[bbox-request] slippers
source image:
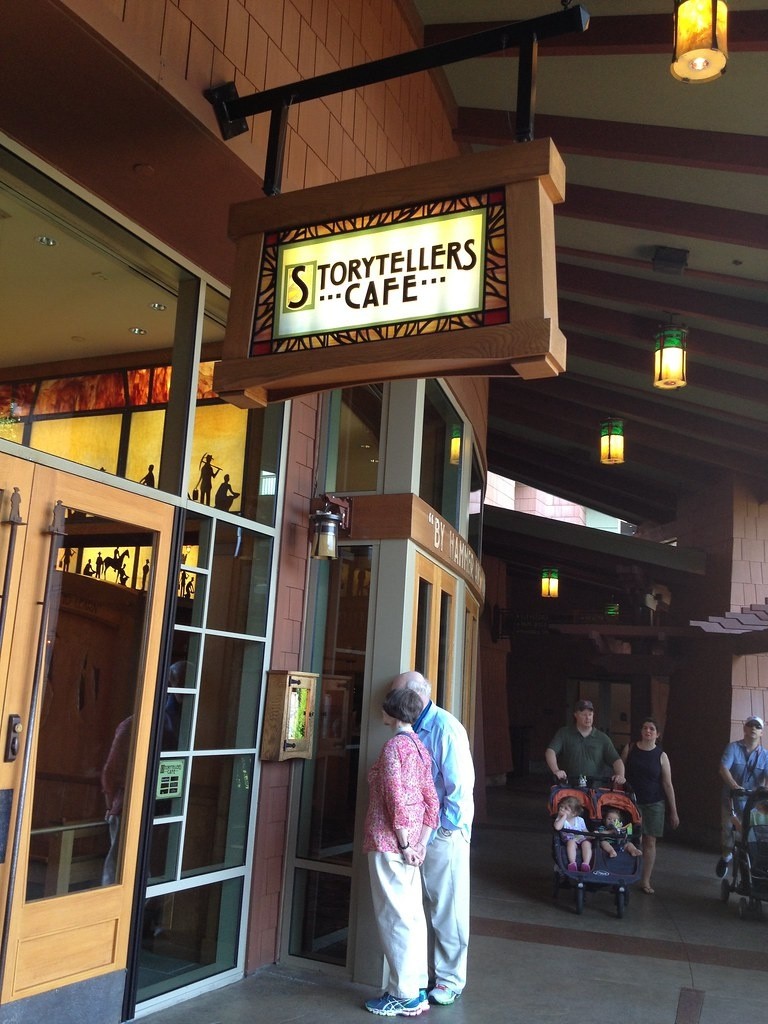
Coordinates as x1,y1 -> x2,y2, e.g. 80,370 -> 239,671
642,883 -> 655,896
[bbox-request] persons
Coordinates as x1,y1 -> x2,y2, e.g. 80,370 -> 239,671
599,810 -> 642,857
545,700 -> 626,789
615,717 -> 680,894
553,796 -> 592,872
392,671 -> 476,1004
716,716 -> 768,877
99,654 -> 201,941
730,787 -> 768,830
361,688 -> 440,1017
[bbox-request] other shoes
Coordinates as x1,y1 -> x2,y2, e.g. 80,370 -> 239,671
580,862 -> 591,873
568,862 -> 577,872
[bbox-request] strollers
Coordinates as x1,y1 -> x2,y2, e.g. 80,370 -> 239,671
548,772 -> 643,919
722,786 -> 767,921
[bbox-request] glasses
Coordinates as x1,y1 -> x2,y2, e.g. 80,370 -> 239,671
745,722 -> 762,729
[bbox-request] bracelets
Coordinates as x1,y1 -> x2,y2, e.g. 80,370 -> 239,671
398,842 -> 409,849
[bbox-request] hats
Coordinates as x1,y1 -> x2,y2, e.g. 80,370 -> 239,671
745,716 -> 764,729
575,700 -> 594,712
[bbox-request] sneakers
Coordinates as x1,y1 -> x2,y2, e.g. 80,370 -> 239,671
363,983 -> 431,1016
429,982 -> 461,1005
716,853 -> 731,878
